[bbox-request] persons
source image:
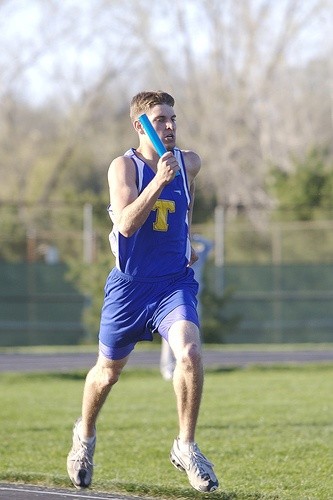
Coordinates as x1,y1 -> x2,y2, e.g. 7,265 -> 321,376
63,92 -> 222,493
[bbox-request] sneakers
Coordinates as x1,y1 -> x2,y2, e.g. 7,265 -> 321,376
169,437 -> 219,492
66,420 -> 96,489
